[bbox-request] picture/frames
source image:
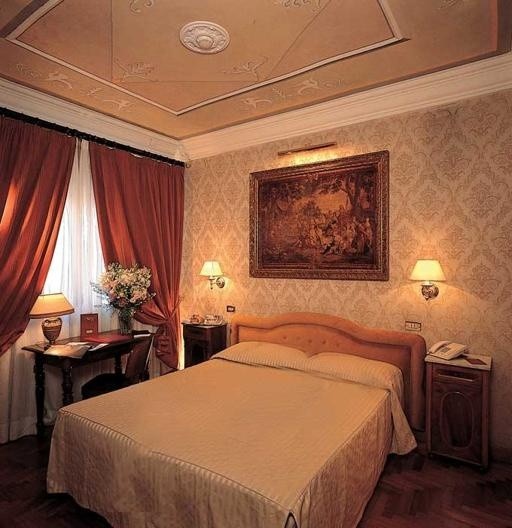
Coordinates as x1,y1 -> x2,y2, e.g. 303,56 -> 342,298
247,149 -> 391,283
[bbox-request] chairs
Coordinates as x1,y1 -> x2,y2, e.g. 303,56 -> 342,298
80,336 -> 153,399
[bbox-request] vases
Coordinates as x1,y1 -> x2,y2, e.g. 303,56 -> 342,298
116,309 -> 136,334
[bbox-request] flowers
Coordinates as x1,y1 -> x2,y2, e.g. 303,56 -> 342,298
88,262 -> 158,333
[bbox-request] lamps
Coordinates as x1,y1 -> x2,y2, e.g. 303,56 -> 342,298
28,291 -> 72,345
408,259 -> 447,302
200,260 -> 227,290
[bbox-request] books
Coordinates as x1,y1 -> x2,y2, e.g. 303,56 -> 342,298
131,330 -> 152,338
43,341 -> 109,359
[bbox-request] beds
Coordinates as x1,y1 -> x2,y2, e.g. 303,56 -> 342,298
57,310 -> 425,528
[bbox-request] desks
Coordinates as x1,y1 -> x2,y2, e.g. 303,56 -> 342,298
21,327 -> 153,434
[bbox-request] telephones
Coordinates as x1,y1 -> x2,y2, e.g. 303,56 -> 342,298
428,341 -> 467,360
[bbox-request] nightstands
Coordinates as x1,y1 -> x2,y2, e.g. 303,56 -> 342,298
181,321 -> 228,369
424,351 -> 494,472
